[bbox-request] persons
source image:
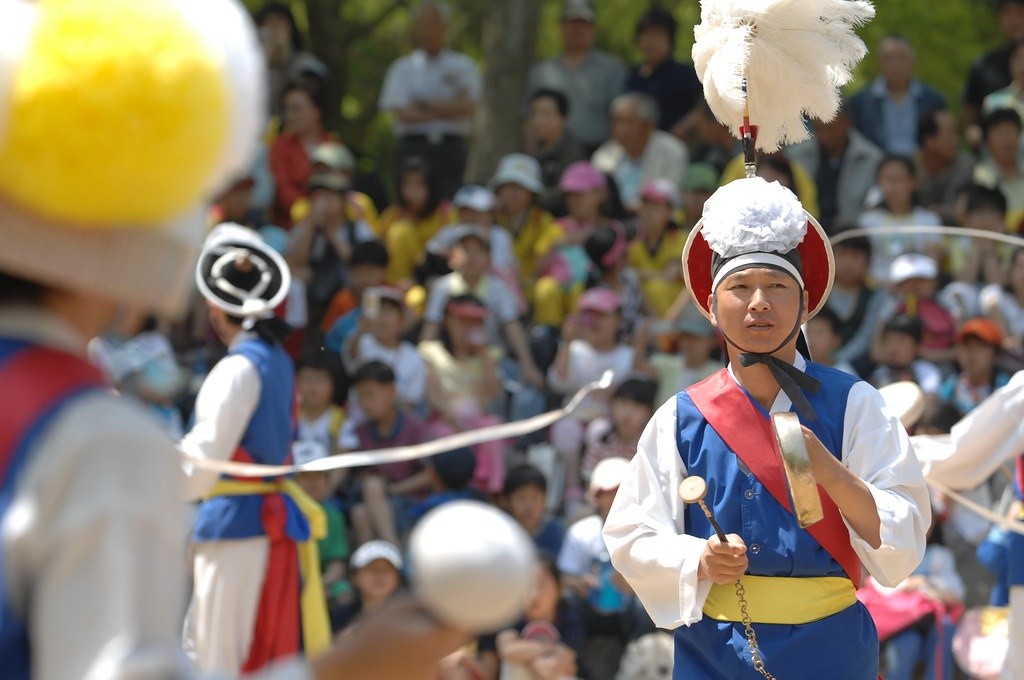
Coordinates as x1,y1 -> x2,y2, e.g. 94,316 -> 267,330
175,222 -> 310,675
90,0 -> 1024,680
600,177 -> 933,680
0,0 -> 479,680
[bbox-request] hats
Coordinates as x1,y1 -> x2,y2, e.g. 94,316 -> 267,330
196,222 -> 291,329
453,184 -> 495,212
890,254 -> 937,284
589,457 -> 630,498
559,161 -> 609,195
640,177 -> 680,212
680,0 -> 875,321
958,317 -> 1004,347
350,540 -> 403,571
0,0 -> 263,319
490,154 -> 544,199
579,290 -> 622,314
563,0 -> 599,26
885,312 -> 923,342
